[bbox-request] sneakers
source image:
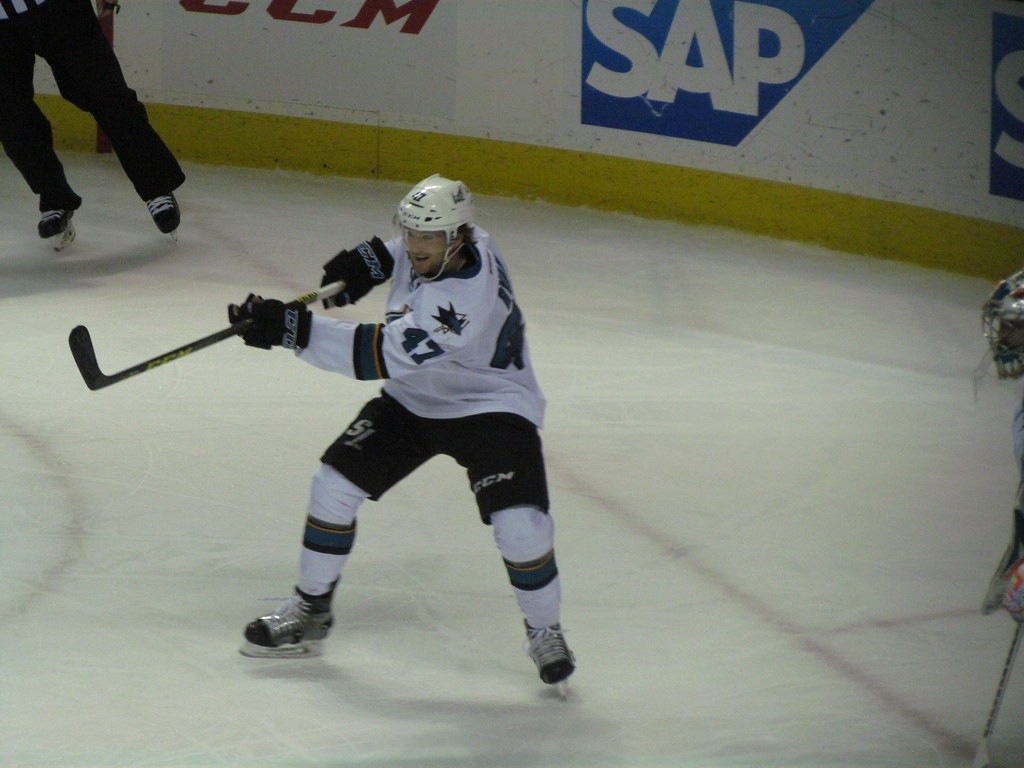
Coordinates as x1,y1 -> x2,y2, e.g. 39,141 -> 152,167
37,208 -> 76,252
239,577 -> 340,659
145,193 -> 181,242
521,620 -> 575,699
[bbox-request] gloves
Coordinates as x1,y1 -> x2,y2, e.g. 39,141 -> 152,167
229,293 -> 313,350
998,541 -> 1024,629
321,234 -> 394,309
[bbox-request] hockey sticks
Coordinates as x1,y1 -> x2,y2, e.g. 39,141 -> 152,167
972,622 -> 1023,768
67,278 -> 350,394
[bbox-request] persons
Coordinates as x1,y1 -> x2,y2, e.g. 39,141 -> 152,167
228,174 -> 575,700
0,0 -> 185,253
981,272 -> 1024,623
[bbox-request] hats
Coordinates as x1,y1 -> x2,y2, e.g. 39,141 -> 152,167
393,173 -> 476,262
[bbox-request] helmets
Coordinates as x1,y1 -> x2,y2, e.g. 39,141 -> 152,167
981,269 -> 1024,379
